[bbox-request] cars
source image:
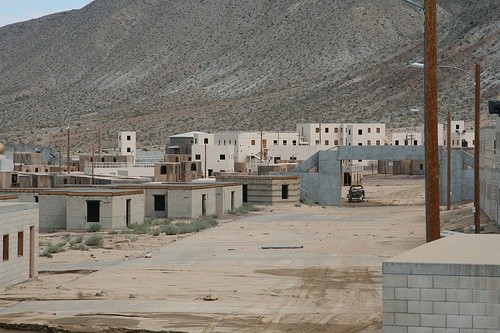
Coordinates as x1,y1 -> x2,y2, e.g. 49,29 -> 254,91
348,184 -> 365,198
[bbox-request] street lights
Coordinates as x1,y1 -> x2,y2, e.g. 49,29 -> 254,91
410,106 -> 453,212
407,61 -> 484,234
